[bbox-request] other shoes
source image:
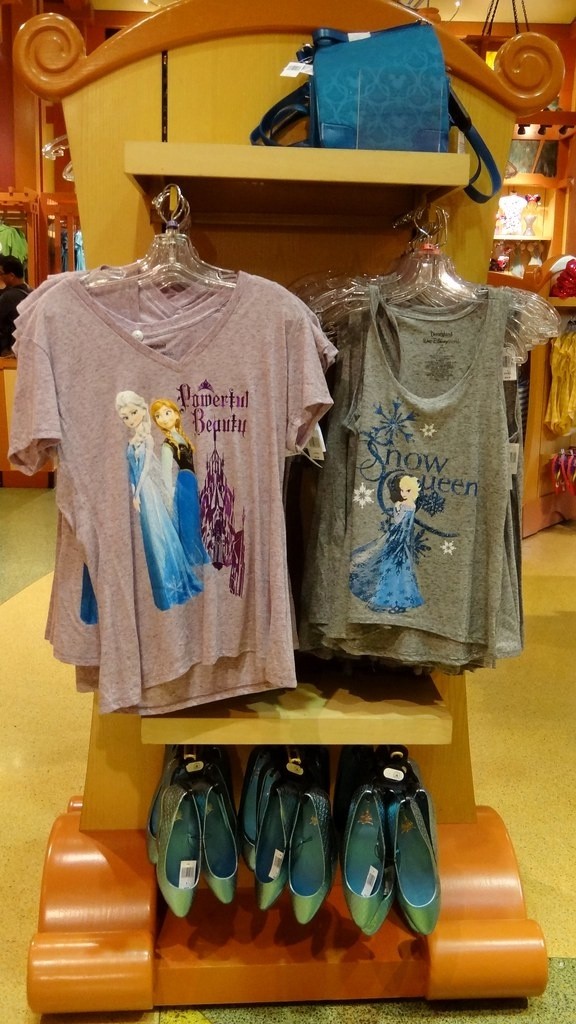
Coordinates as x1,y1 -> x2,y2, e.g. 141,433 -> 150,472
147,746 -> 443,939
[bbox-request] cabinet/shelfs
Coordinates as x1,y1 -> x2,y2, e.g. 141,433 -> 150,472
120,138 -> 472,751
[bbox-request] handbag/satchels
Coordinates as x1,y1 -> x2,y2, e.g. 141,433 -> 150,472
251,18 -> 502,203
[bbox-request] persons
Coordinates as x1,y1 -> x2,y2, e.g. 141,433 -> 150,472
1,254 -> 36,359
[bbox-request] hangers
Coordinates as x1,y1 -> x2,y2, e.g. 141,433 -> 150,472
76,182 -> 568,367
